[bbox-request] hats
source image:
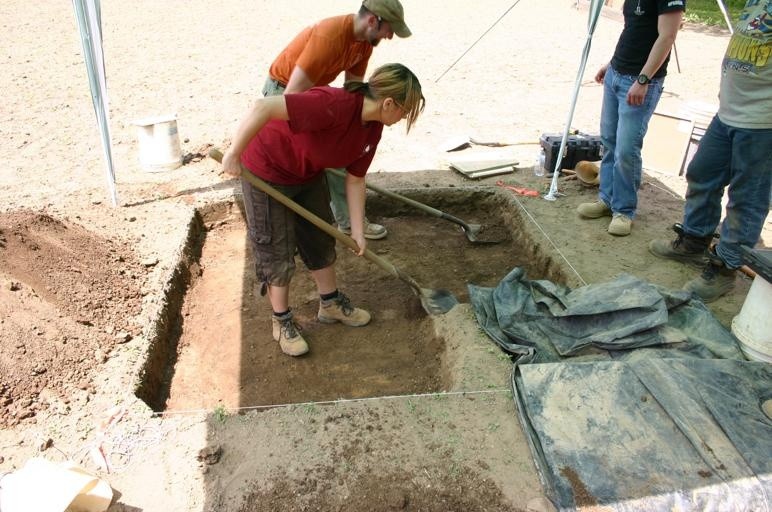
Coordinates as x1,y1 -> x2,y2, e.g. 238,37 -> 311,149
362,0 -> 412,37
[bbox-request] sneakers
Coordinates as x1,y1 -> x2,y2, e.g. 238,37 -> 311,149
272,312 -> 309,356
683,254 -> 737,303
578,200 -> 613,217
649,223 -> 714,269
318,293 -> 371,327
336,217 -> 387,239
607,212 -> 633,236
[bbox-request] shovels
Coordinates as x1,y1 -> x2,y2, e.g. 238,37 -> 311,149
322,167 -> 509,244
208,147 -> 458,316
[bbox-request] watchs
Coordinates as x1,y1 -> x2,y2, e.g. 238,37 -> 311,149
636,74 -> 651,85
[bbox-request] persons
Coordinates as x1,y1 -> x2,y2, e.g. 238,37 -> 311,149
262,0 -> 413,239
221,64 -> 426,356
646,0 -> 772,303
576,0 -> 685,236
